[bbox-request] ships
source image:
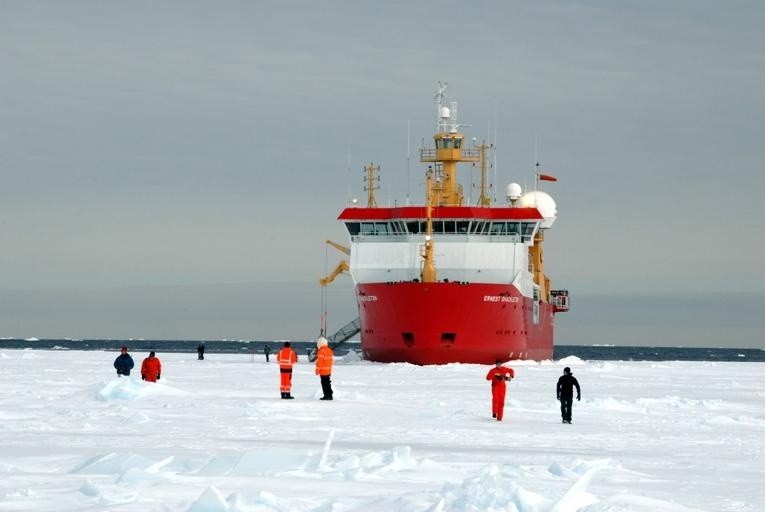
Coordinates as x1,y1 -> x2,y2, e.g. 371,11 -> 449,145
306,80 -> 569,364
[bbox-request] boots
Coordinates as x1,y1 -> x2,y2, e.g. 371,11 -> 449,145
320,392 -> 332,400
281,393 -> 294,399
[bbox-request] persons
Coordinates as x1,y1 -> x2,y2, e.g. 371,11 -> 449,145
201,345 -> 204,360
556,367 -> 580,424
140,351 -> 161,382
486,359 -> 514,421
277,342 -> 296,399
264,345 -> 271,362
197,344 -> 201,360
114,345 -> 134,376
315,336 -> 333,400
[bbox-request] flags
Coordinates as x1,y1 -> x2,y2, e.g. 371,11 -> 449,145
537,174 -> 557,181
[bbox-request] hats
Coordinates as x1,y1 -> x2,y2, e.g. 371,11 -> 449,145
284,342 -> 290,347
495,359 -> 502,364
150,352 -> 155,357
121,347 -> 127,352
564,367 -> 570,373
